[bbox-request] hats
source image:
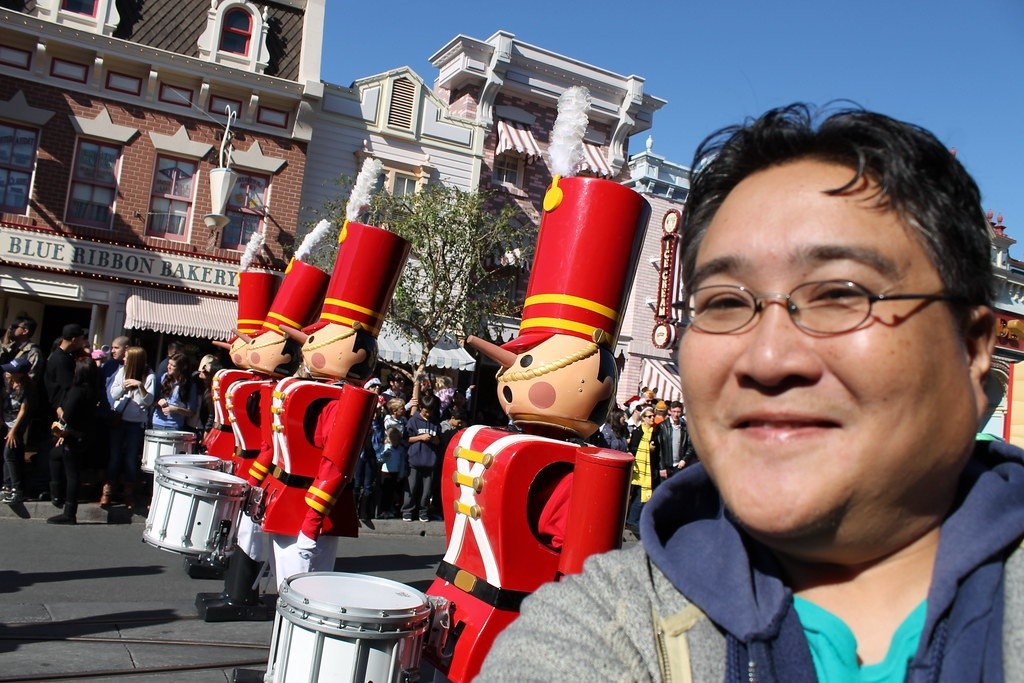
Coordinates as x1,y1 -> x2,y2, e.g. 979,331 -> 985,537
656,401 -> 667,411
0,357 -> 31,374
62,324 -> 89,340
361,374 -> 381,389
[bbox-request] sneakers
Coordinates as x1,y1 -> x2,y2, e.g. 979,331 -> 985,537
418,515 -> 429,522
403,514 -> 412,522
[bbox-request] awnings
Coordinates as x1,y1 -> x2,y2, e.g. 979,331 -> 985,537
639,358 -> 686,420
495,115 -> 542,165
376,319 -> 476,371
124,286 -> 238,342
577,141 -> 614,177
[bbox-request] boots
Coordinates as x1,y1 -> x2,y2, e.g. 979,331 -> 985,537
46,481 -> 63,506
358,494 -> 372,523
46,502 -> 77,525
124,482 -> 134,510
99,480 -> 113,507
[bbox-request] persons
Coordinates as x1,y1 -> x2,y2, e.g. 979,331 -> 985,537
195,256 -> 332,622
233,221 -> 411,683
471,102 -> 1023,683
353,371 -> 476,522
184,272 -> 282,580
425,175 -> 652,683
586,388 -> 699,535
0,314 -> 223,525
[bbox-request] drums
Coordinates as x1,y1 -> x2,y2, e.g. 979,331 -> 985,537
140,429 -> 196,474
263,571 -> 433,683
153,454 -> 224,499
142,463 -> 251,560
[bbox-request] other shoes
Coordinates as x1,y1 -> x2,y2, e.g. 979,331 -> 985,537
0,486 -> 12,495
626,524 -> 641,532
379,511 -> 394,519
3,488 -> 23,503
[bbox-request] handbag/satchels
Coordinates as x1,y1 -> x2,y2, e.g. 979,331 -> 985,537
102,409 -> 122,429
51,423 -> 71,437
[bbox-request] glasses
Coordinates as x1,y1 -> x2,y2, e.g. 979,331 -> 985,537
10,324 -> 25,330
644,415 -> 655,418
670,277 -> 974,336
